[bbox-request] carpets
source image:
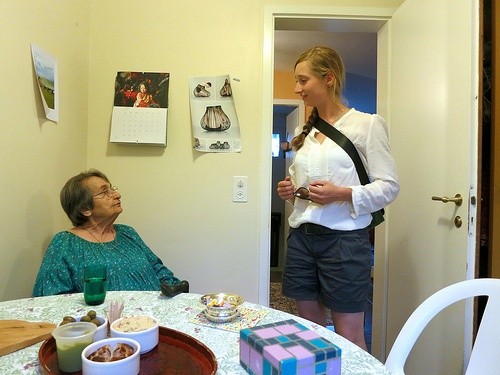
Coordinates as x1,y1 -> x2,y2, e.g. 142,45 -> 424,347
268,283 -> 335,326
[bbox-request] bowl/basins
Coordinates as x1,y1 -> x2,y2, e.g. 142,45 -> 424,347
109,314 -> 160,356
81,337 -> 141,375
201,291 -> 245,323
57,316 -> 108,342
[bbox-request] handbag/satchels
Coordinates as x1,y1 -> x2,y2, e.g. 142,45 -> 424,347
313,118 -> 385,231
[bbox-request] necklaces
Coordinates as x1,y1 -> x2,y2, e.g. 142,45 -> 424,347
84,229 -> 118,250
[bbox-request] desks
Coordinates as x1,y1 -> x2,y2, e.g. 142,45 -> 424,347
0,291 -> 391,375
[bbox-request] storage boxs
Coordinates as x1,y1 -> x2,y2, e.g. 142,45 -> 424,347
239,319 -> 342,375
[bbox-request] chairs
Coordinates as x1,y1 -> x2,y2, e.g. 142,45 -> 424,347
383,278 -> 500,375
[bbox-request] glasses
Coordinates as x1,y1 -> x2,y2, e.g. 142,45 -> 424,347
94,185 -> 119,197
294,187 -> 314,203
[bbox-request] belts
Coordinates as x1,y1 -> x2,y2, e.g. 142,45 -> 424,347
296,223 -> 332,236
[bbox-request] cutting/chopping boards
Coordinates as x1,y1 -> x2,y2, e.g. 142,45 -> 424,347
0,318 -> 58,356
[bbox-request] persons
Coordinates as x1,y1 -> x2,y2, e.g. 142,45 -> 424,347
276,47 -> 400,353
32,168 -> 180,298
134,81 -> 157,108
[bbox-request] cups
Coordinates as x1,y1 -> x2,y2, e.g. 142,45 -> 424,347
51,322 -> 97,373
104,306 -> 125,337
83,264 -> 107,305
